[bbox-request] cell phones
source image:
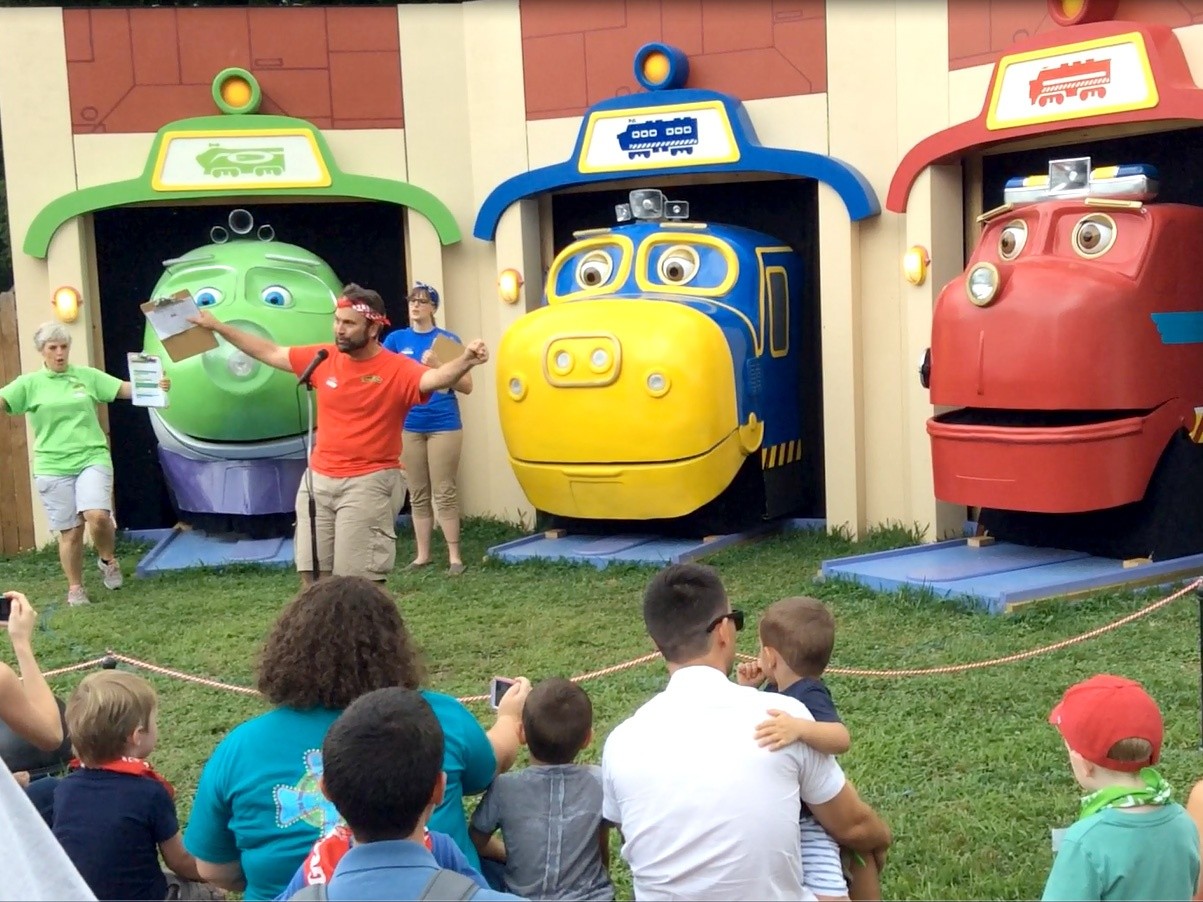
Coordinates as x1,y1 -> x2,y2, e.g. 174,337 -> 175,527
0,596 -> 12,624
489,678 -> 517,709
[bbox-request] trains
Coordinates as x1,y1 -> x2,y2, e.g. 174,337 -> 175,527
142,207 -> 347,517
913,154 -> 1203,563
498,185 -> 825,534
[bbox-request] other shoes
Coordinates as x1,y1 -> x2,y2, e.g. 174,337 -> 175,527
448,563 -> 467,577
399,561 -> 432,572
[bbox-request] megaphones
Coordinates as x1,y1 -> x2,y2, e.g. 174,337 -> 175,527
664,200 -> 689,219
1048,157 -> 1091,195
614,204 -> 633,221
629,189 -> 666,219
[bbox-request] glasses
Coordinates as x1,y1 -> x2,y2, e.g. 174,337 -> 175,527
408,298 -> 435,306
707,609 -> 745,633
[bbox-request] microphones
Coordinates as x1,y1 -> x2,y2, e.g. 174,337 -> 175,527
298,349 -> 328,385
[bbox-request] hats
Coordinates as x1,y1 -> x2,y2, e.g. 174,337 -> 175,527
1048,674 -> 1165,771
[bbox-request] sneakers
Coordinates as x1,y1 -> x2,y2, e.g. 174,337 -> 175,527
97,557 -> 123,590
66,589 -> 90,606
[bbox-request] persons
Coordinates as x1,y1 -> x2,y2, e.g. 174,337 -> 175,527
602,563 -> 891,902
180,575 -> 533,902
184,284 -> 490,587
1040,676 -> 1203,902
0,590 -> 227,902
0,321 -> 172,608
379,284 -> 473,577
469,676 -> 619,902
